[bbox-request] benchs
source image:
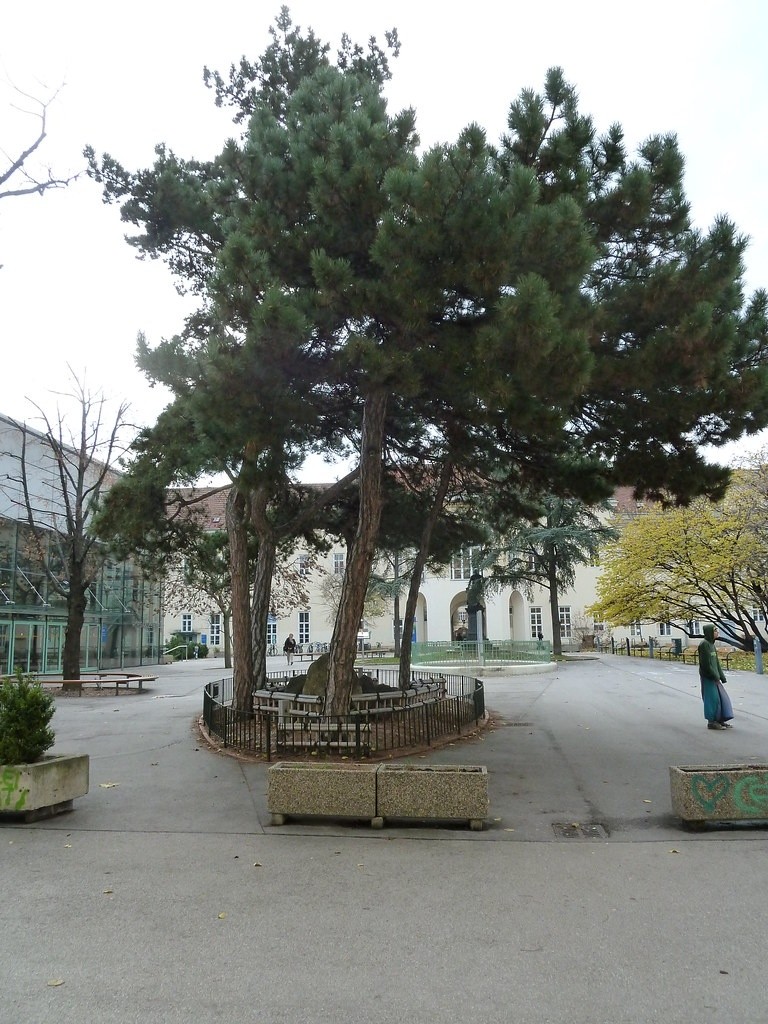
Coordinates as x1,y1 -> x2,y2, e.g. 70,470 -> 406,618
357,650 -> 388,658
599,643 -> 735,670
1,672 -> 158,696
294,651 -> 328,662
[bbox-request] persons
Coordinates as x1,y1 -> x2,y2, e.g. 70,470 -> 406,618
697,624 -> 734,729
538,630 -> 545,649
456,633 -> 463,647
468,568 -> 483,605
285,634 -> 296,666
195,643 -> 199,660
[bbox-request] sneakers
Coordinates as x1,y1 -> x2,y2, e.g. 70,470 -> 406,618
718,721 -> 733,727
707,720 -> 726,730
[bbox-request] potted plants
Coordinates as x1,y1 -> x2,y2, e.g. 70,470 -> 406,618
0,667 -> 89,823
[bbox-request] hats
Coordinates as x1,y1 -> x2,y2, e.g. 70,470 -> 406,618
714,624 -> 718,631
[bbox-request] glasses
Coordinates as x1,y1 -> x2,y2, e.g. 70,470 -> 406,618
714,628 -> 719,631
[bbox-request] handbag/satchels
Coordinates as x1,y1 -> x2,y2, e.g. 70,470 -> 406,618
283,646 -> 288,652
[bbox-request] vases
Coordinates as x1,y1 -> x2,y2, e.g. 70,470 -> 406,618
266,761 -> 489,831
668,763 -> 768,832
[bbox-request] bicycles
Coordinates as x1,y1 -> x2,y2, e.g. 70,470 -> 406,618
266,640 -> 383,658
